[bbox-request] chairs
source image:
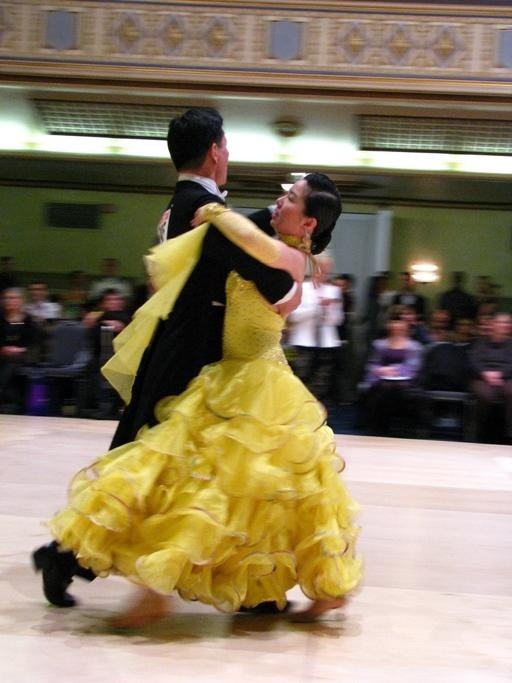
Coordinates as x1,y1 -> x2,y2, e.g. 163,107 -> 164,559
418,340 -> 475,439
19,322 -> 95,415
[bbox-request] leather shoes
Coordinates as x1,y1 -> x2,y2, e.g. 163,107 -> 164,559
233,595 -> 292,617
32,541 -> 78,608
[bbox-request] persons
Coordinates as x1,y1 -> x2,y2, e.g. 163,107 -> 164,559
41,170 -> 367,628
32,105 -> 304,616
0,255 -> 512,445
